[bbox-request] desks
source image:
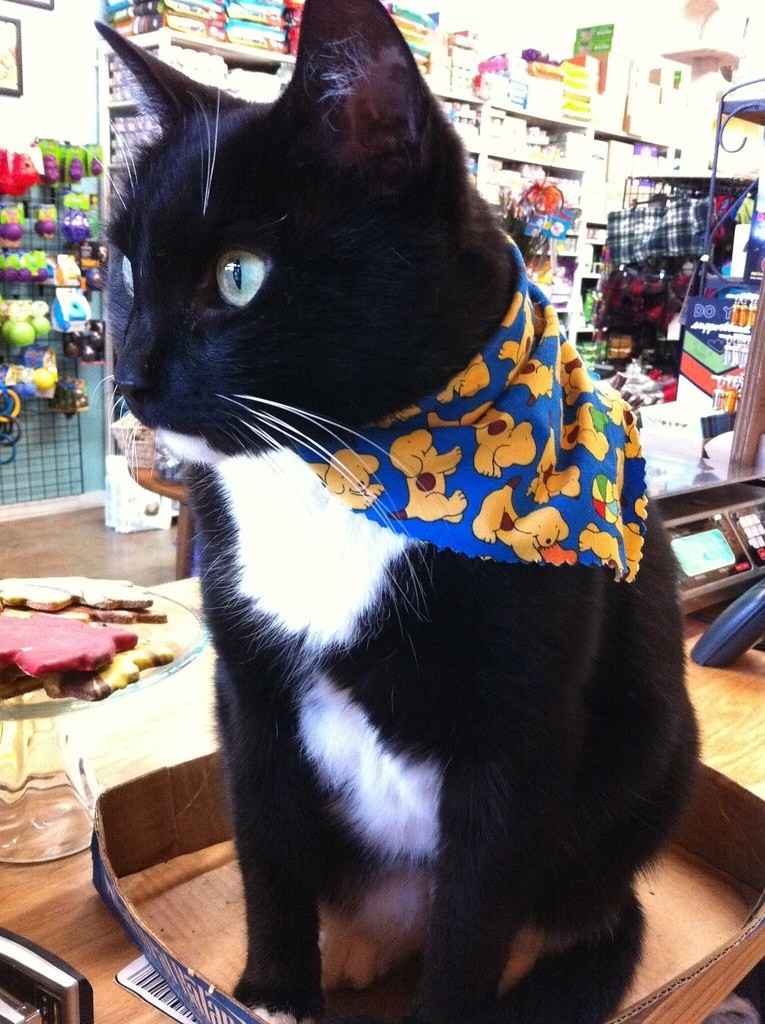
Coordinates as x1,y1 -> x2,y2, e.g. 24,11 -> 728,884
128,467 -> 194,581
0,781 -> 764,1024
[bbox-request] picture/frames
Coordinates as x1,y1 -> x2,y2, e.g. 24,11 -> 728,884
0,15 -> 24,97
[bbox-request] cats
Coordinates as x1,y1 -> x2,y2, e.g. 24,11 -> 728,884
95,1 -> 706,1024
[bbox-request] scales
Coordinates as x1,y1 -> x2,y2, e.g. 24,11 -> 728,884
640,437 -> 765,616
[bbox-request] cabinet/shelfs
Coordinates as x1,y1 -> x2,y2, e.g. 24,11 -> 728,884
98,0 -> 765,581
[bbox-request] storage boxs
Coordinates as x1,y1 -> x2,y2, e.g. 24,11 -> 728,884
473,20 -> 698,144
93,750 -> 765,1024
